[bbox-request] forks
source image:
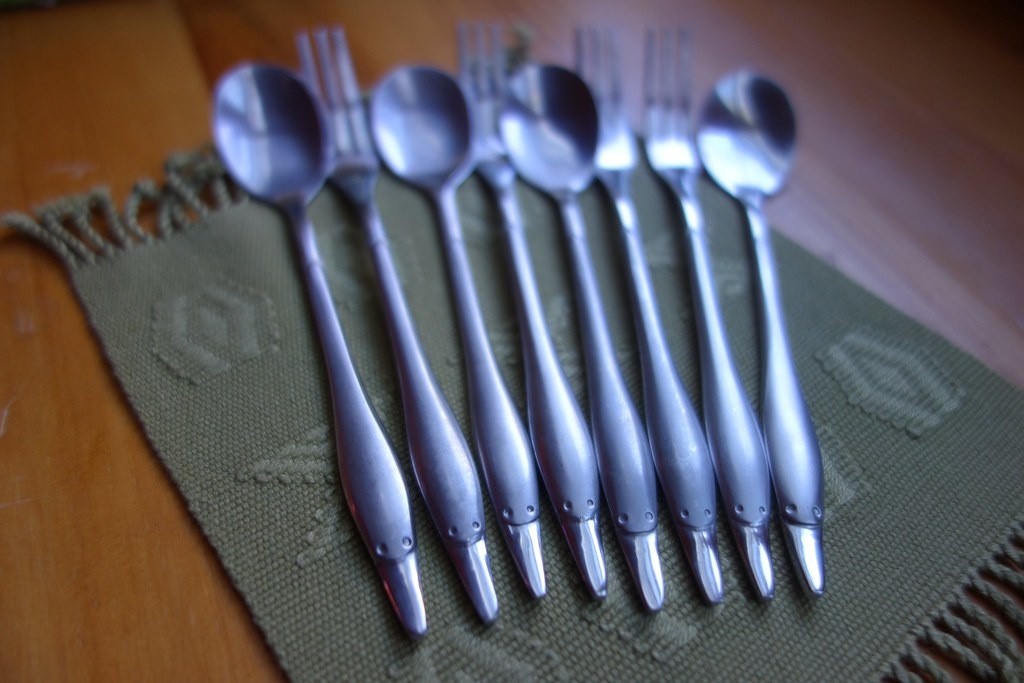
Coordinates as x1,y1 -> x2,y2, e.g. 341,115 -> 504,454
573,28 -> 724,602
643,28 -> 774,602
295,28 -> 499,624
456,24 -> 610,600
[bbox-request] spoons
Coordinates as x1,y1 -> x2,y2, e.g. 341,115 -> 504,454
370,67 -> 548,601
695,73 -> 827,595
213,64 -> 428,637
494,64 -> 665,611
